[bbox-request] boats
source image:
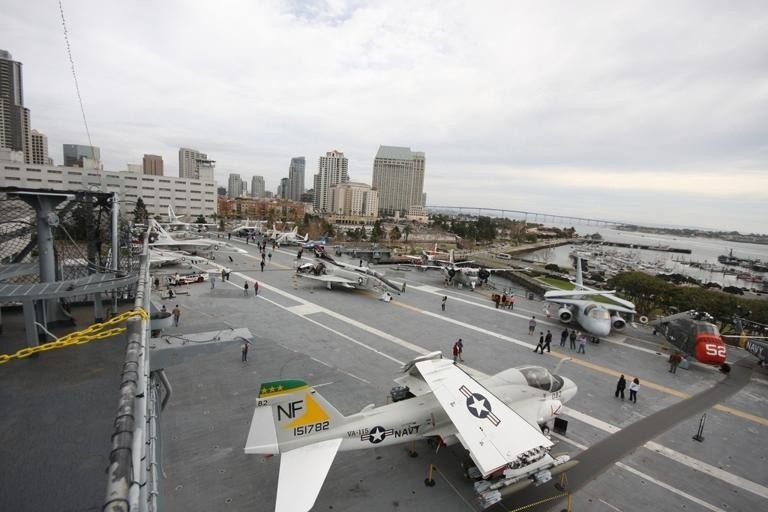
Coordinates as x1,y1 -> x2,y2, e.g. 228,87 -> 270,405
568,236 -> 767,297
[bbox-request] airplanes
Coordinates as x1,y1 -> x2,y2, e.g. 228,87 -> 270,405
401,242 -> 536,290
242,350 -> 582,512
544,252 -> 639,345
719,319 -> 767,368
290,252 -> 408,301
116,203 -> 330,272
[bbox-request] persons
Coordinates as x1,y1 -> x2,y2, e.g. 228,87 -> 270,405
160,305 -> 167,312
169,288 -> 173,298
672,351 -> 681,374
457,338 -> 463,360
528,315 -> 537,334
441,295 -> 448,311
495,293 -> 500,308
242,235 -> 280,272
240,342 -> 249,363
453,341 -> 460,361
243,280 -> 249,295
402,281 -> 406,292
297,248 -> 303,258
532,327 -> 587,355
254,282 -> 259,295
508,295 -> 515,310
228,231 -> 231,239
359,258 -> 362,266
171,305 -> 180,325
501,294 -> 506,309
615,374 -> 627,399
668,350 -> 678,372
175,271 -> 180,283
628,378 -> 640,404
211,274 -> 215,288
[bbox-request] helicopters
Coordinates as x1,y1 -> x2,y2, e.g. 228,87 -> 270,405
639,299 -> 753,378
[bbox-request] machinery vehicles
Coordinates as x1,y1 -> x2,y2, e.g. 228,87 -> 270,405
164,270 -> 210,286
